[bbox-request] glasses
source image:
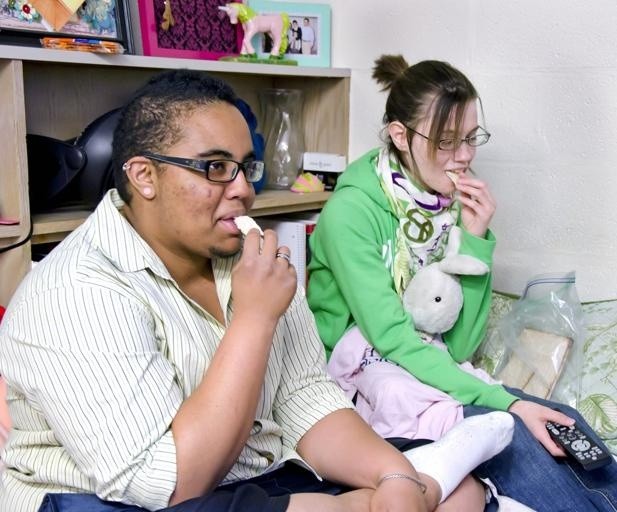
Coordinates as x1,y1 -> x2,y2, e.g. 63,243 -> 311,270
142,151 -> 266,184
402,123 -> 491,151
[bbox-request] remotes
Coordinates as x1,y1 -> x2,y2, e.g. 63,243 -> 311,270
546,407 -> 612,471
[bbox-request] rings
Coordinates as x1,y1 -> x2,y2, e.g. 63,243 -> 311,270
276,252 -> 290,261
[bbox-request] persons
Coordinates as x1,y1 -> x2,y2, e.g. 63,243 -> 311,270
1,68 -> 517,510
305,53 -> 617,510
290,20 -> 301,53
301,18 -> 315,54
263,22 -> 293,53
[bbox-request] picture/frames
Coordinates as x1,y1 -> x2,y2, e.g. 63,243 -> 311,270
249,0 -> 330,68
0,0 -> 136,55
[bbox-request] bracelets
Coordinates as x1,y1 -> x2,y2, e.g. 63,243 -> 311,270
375,473 -> 429,493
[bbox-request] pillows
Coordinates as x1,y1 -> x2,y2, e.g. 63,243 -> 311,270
472,290 -> 617,462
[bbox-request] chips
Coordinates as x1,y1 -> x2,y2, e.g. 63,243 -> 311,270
446,171 -> 460,184
234,216 -> 264,235
